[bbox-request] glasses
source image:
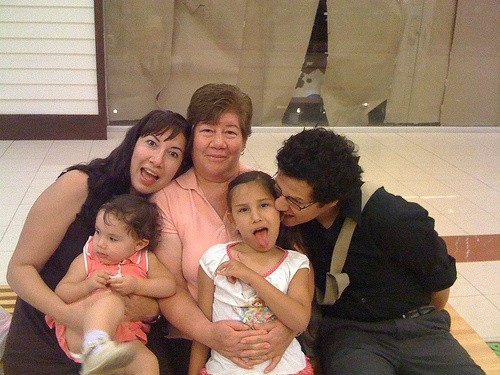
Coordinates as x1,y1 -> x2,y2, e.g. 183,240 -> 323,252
265,172 -> 320,211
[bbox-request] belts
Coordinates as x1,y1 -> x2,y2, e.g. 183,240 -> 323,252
392,306 -> 434,321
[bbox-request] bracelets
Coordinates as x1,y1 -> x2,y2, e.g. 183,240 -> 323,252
294,331 -> 304,336
145,311 -> 162,325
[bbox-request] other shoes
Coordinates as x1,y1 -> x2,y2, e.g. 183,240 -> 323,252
78,339 -> 144,375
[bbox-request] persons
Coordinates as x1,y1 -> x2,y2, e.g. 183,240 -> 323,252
3,110 -> 194,375
189,170 -> 316,375
272,126 -> 488,375
42,192 -> 178,375
142,83 -> 278,375
